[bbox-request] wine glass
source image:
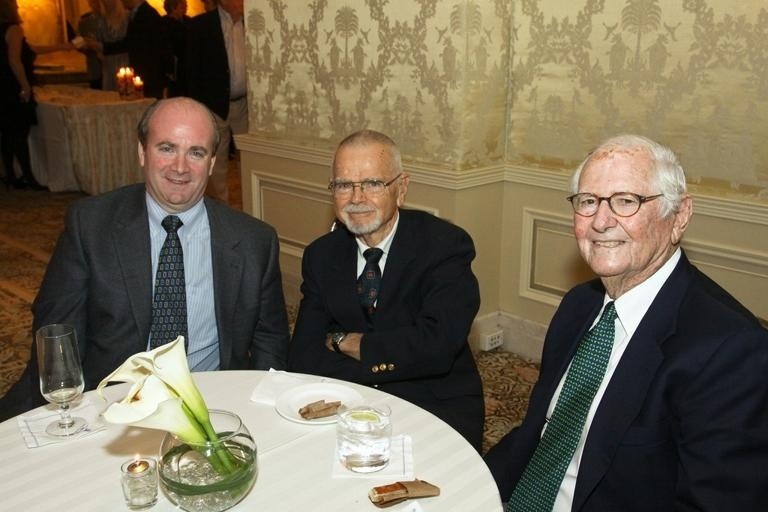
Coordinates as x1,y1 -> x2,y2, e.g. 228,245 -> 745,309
36,324 -> 88,436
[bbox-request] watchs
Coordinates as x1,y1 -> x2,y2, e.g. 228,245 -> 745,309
331,329 -> 350,351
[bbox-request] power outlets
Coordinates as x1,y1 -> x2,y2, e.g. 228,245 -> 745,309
481,329 -> 504,352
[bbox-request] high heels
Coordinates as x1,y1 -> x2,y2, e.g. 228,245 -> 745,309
18,175 -> 49,192
2,176 -> 18,190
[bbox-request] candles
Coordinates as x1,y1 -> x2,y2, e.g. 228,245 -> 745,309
127,452 -> 149,473
132,76 -> 144,99
125,67 -> 134,94
116,67 -> 126,96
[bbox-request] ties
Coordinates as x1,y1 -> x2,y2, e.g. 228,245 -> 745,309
505,300 -> 619,512
357,248 -> 384,316
149,215 -> 188,355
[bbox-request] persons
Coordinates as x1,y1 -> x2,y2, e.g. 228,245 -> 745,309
0,96 -> 285,425
0,0 -> 50,194
76,1 -> 248,206
288,129 -> 487,453
481,133 -> 768,512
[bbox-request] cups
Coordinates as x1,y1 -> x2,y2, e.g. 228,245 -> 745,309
337,401 -> 392,473
121,458 -> 158,511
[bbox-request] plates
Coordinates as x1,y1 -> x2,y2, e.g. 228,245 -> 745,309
275,382 -> 364,425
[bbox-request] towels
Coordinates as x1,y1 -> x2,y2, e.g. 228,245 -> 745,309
331,406 -> 416,482
16,400 -> 109,449
249,367 -> 310,407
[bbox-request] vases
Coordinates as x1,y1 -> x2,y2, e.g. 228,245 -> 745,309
159,407 -> 256,512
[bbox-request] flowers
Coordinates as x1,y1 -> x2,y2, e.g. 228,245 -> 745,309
97,334 -> 255,494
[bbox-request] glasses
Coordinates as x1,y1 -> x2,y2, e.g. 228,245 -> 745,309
327,173 -> 403,194
566,191 -> 664,218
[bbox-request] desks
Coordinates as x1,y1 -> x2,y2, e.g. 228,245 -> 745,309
32,49 -> 92,86
